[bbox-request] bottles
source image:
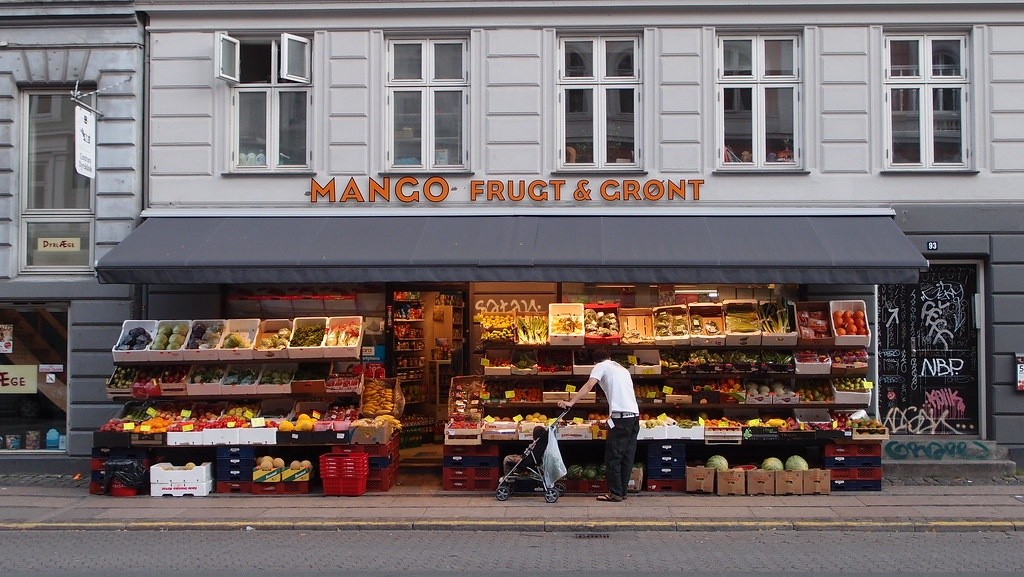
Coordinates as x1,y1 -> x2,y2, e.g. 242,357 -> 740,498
247,152 -> 256,165
256,150 -> 266,166
240,152 -> 247,166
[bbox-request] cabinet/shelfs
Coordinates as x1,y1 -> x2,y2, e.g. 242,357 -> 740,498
394,299 -> 429,404
434,305 -> 464,350
428,360 -> 451,407
479,342 -> 881,448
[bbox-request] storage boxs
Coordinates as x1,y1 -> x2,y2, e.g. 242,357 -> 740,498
256,363 -> 298,394
360,377 -> 406,418
395,131 -> 421,138
132,362 -> 167,396
130,400 -> 176,445
276,401 -> 330,444
221,364 -> 264,395
158,361 -> 195,396
442,299 -> 890,496
349,423 -> 394,444
311,402 -> 359,443
202,399 -> 261,445
105,362 -> 146,400
186,361 -> 230,396
291,361 -> 332,393
167,401 -> 228,446
89,431 -> 401,498
93,401 -> 152,448
112,316 -> 365,361
239,398 -> 297,445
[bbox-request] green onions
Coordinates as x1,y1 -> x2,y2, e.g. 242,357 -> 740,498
760,295 -> 789,334
518,318 -> 547,346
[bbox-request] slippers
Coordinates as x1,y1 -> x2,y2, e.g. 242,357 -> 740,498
597,495 -> 627,501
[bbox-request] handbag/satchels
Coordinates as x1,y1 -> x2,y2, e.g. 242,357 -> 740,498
543,426 -> 567,488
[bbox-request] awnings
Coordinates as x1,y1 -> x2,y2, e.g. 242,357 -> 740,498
93,215 -> 929,285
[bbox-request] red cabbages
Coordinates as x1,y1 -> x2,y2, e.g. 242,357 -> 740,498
117,327 -> 152,350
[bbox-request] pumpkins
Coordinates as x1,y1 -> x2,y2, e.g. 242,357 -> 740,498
256,457 -> 311,471
278,414 -> 317,431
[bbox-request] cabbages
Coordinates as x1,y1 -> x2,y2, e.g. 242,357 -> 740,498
151,324 -> 188,350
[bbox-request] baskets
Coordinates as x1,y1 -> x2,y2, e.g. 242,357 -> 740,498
216,447 -> 256,493
251,482 -> 311,494
644,444 -> 686,492
510,481 -> 609,493
442,442 -> 504,491
821,444 -> 881,491
332,431 -> 400,491
320,453 -> 368,496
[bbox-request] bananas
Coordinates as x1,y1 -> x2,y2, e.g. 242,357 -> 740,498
353,382 -> 402,432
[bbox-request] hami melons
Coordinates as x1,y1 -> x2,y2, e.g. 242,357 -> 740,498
747,382 -> 794,396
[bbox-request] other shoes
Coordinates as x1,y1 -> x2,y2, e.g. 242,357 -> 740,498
499,477 -> 504,482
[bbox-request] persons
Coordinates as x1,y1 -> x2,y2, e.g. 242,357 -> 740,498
499,426 -> 547,482
557,348 -> 639,503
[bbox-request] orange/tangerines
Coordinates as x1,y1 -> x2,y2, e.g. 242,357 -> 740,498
834,311 -> 866,335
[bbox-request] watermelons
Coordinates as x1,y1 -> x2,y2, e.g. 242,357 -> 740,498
707,455 -> 728,471
569,459 -> 606,481
786,455 -> 808,470
761,458 -> 783,471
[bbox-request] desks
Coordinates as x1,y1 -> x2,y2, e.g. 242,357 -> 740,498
239,136 -> 306,165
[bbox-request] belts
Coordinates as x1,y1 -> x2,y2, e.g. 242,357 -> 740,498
607,412 -> 639,429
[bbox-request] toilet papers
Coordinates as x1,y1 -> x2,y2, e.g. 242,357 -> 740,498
850,409 -> 870,425
